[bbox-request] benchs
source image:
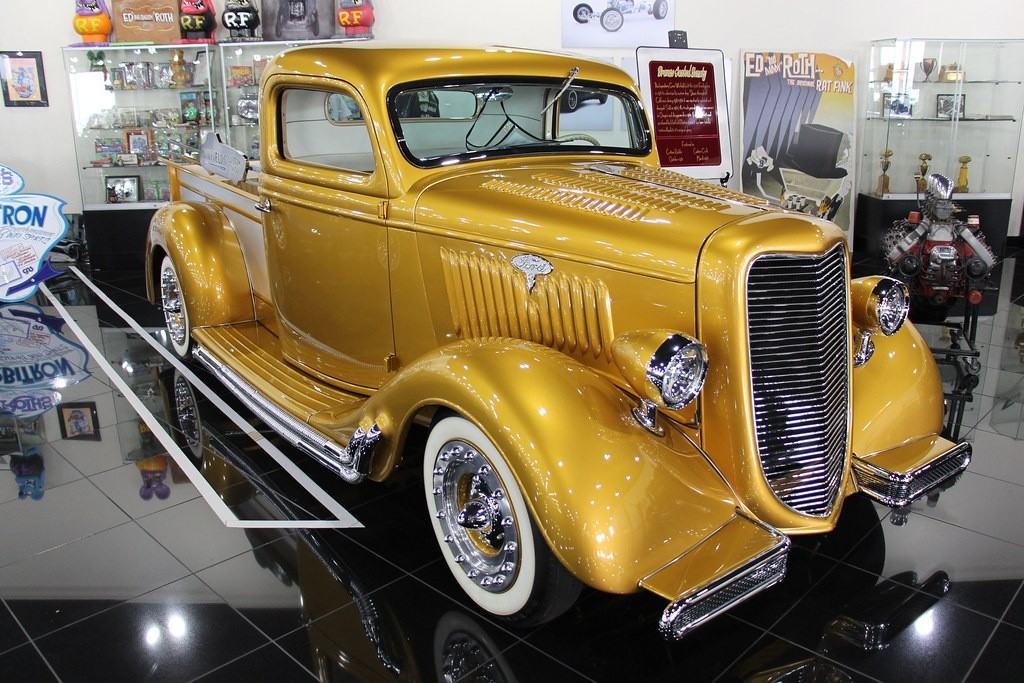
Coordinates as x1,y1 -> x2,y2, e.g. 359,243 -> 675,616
294,147 -> 485,171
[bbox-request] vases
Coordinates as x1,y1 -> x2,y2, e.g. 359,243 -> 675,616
57,401 -> 102,442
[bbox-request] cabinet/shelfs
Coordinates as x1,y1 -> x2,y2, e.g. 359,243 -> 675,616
219,39 -> 366,160
851,40 -> 1024,316
62,37 -> 224,329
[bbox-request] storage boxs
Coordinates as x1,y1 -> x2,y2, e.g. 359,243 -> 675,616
117,154 -> 138,164
226,59 -> 270,86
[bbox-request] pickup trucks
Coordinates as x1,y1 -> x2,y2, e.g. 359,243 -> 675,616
143,40 -> 973,646
79,333 -> 950,682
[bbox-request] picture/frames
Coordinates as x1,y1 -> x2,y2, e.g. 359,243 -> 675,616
105,175 -> 139,203
0,50 -> 49,108
191,49 -> 216,87
124,130 -> 155,162
